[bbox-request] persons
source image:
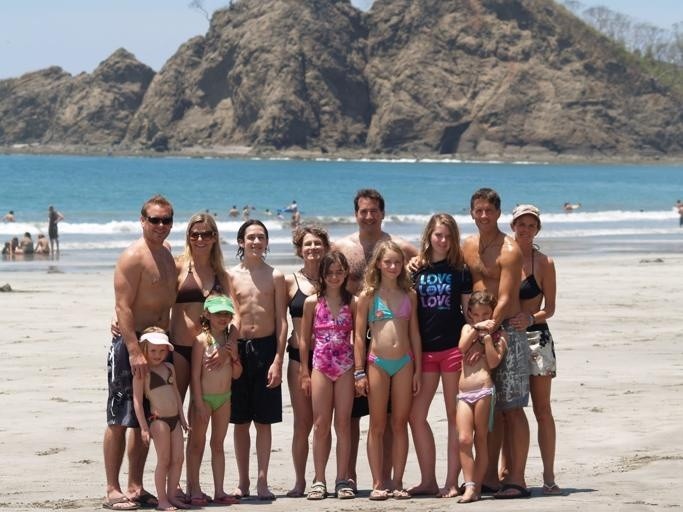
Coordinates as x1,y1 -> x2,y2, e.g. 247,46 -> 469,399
224,220 -> 288,499
134,327 -> 189,510
103,195 -> 179,509
409,213 -> 473,498
111,213 -> 241,502
284,224 -> 328,498
338,190 -> 417,496
16,233 -> 35,254
49,205 -> 64,251
354,240 -> 421,499
188,295 -> 243,505
2,237 -> 18,256
230,201 -> 299,224
407,188 -> 531,498
301,251 -> 359,499
458,291 -> 505,503
499,205 -> 561,494
36,235 -> 50,254
3,211 -> 16,222
565,202 -> 582,210
676,199 -> 683,226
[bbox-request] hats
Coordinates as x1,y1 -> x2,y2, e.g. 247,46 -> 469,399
203,296 -> 234,314
512,204 -> 541,223
138,332 -> 174,351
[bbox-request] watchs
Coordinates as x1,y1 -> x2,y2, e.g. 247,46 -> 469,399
530,313 -> 536,322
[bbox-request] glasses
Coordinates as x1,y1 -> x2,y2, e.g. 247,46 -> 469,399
189,230 -> 217,241
145,216 -> 172,225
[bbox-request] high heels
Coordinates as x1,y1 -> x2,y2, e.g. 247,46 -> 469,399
235,486 -> 250,499
346,478 -> 358,497
257,485 -> 276,501
287,478 -> 306,498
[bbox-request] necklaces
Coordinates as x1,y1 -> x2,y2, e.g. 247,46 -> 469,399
477,231 -> 501,255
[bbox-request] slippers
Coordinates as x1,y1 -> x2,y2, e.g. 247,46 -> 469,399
102,495 -> 140,510
190,495 -> 209,506
130,493 -> 159,507
494,483 -> 532,499
460,483 -> 501,495
369,489 -> 388,500
213,495 -> 239,503
543,483 -> 562,495
390,487 -> 412,500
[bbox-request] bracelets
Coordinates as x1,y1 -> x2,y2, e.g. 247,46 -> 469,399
354,364 -> 366,380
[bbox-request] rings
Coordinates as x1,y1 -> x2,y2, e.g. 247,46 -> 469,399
518,320 -> 522,325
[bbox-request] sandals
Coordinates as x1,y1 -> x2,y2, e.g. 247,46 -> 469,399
185,480 -> 214,504
307,481 -> 327,500
175,482 -> 192,504
334,480 -> 356,499
